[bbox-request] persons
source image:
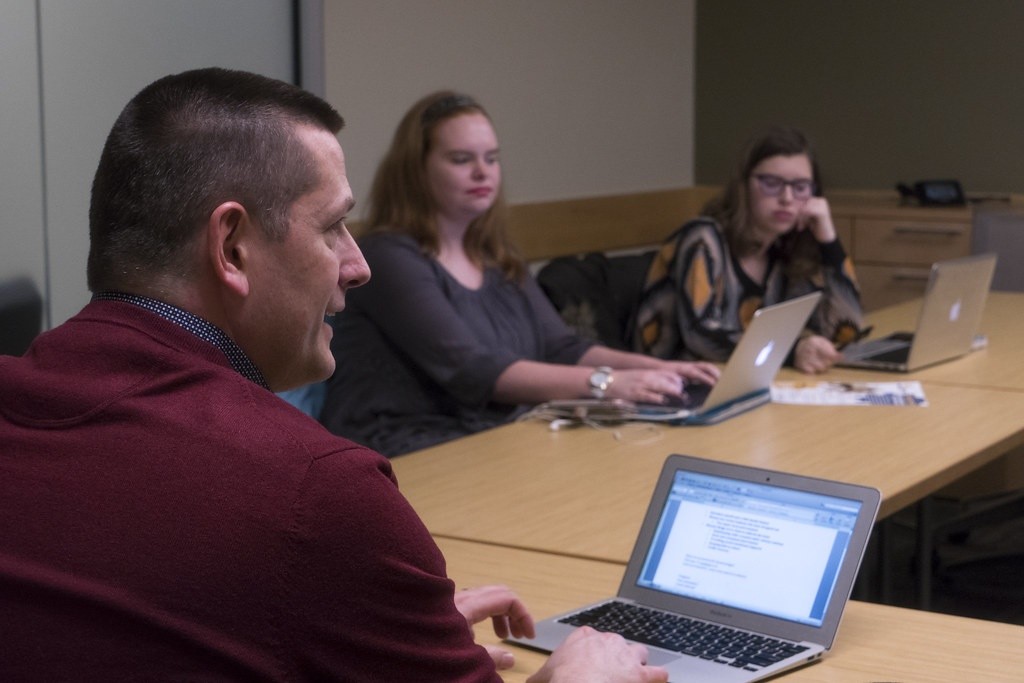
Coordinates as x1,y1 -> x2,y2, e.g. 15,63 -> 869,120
304,88 -> 721,456
626,129 -> 874,374
0,66 -> 670,681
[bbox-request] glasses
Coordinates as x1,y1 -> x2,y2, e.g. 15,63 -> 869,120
752,175 -> 816,200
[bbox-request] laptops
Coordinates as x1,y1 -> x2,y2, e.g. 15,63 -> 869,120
828,253 -> 998,372
503,452 -> 882,683
573,290 -> 824,419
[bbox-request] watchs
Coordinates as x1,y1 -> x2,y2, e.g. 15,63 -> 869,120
588,365 -> 612,398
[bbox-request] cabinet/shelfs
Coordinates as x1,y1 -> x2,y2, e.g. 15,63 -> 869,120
828,209 -> 974,303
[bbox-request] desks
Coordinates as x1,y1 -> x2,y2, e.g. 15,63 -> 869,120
386,289 -> 1024,683
